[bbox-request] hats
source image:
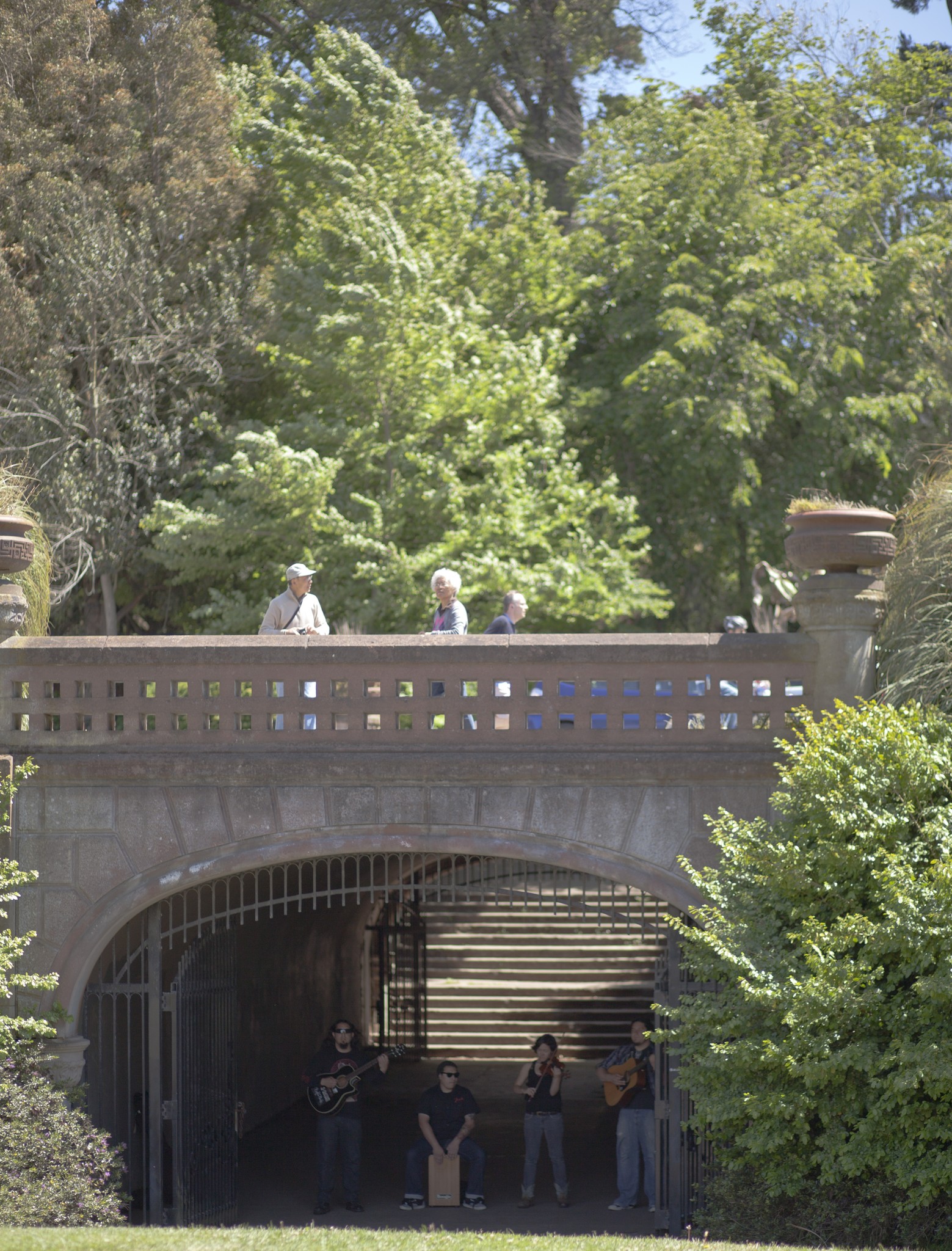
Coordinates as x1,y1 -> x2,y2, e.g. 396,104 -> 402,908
286,563 -> 316,581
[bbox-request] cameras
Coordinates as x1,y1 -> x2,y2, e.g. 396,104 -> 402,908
298,628 -> 307,635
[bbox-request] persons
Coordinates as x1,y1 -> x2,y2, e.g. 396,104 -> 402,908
513,1034 -> 571,1207
695,616 -> 748,730
595,1018 -> 656,1211
302,1019 -> 389,1216
424,568 -> 475,730
258,563 -> 330,729
485,590 -> 528,730
399,1062 -> 486,1210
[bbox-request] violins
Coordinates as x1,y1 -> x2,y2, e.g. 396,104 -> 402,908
540,1056 -> 570,1077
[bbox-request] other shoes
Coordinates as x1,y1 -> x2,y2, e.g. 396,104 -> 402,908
463,1198 -> 486,1210
345,1199 -> 364,1212
314,1202 -> 331,1215
399,1200 -> 425,1210
607,1203 -> 635,1210
649,1204 -> 655,1212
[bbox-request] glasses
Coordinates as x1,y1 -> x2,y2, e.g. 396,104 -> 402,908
335,1028 -> 352,1033
307,575 -> 313,581
434,583 -> 447,587
442,1072 -> 459,1078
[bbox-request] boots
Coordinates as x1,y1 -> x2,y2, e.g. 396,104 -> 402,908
518,1184 -> 534,1208
554,1182 -> 570,1207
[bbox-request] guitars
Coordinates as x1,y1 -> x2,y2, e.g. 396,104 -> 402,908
306,1041 -> 408,1116
605,1055 -> 652,1105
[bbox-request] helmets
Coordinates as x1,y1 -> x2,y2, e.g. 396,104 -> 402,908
723,615 -> 748,631
